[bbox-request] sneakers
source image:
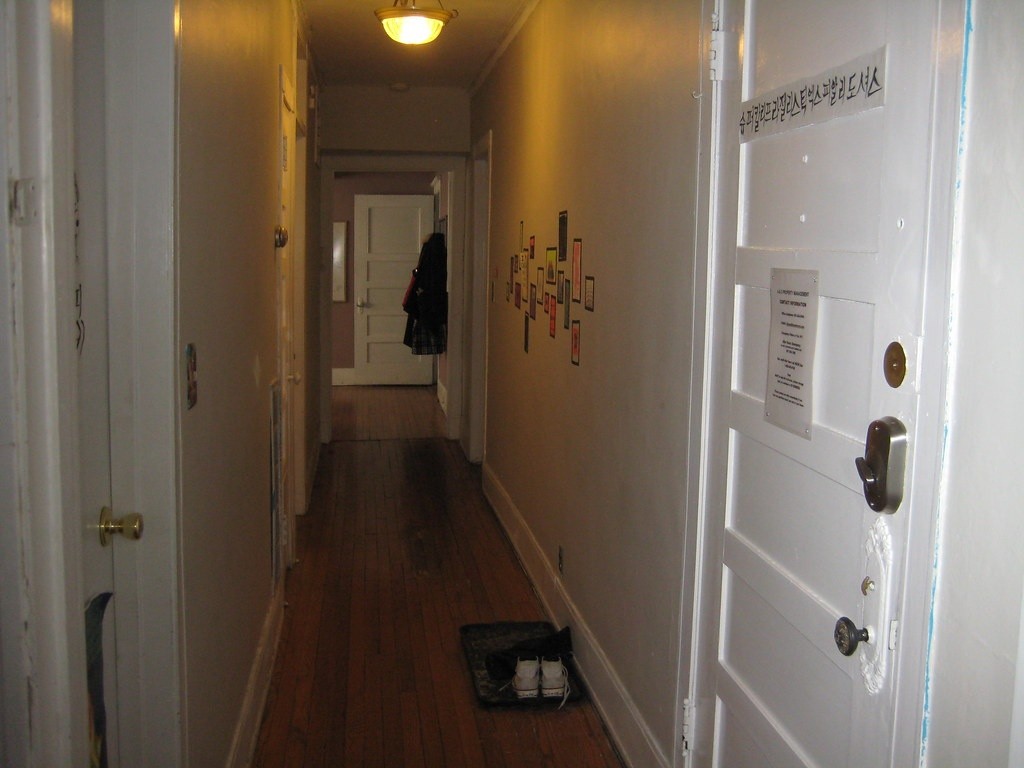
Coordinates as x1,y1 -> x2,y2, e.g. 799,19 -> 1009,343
498,652 -> 540,699
542,656 -> 571,710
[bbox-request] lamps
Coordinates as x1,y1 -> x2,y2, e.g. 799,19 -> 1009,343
374,0 -> 459,44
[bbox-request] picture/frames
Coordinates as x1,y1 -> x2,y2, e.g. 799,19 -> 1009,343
491,211 -> 594,366
